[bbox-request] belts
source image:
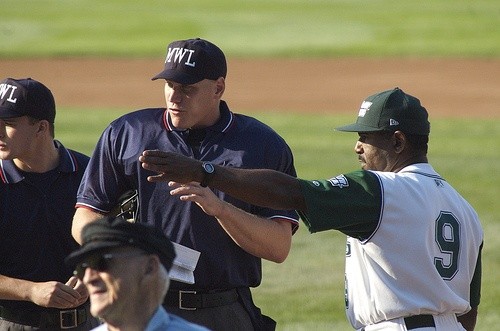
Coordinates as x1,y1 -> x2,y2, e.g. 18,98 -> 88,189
405,314 -> 435,329
0,306 -> 93,329
162,289 -> 238,310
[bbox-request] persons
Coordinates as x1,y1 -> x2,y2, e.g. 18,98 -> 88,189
71,38 -> 299,331
0,77 -> 104,331
66,219 -> 209,331
139,87 -> 484,331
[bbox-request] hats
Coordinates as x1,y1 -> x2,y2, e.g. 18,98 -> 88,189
66,216 -> 176,272
151,38 -> 227,84
333,87 -> 431,135
0,78 -> 56,122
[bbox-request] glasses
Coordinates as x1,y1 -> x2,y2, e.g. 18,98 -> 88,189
73,251 -> 149,279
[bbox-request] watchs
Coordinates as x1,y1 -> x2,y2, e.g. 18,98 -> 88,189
200,161 -> 215,187
